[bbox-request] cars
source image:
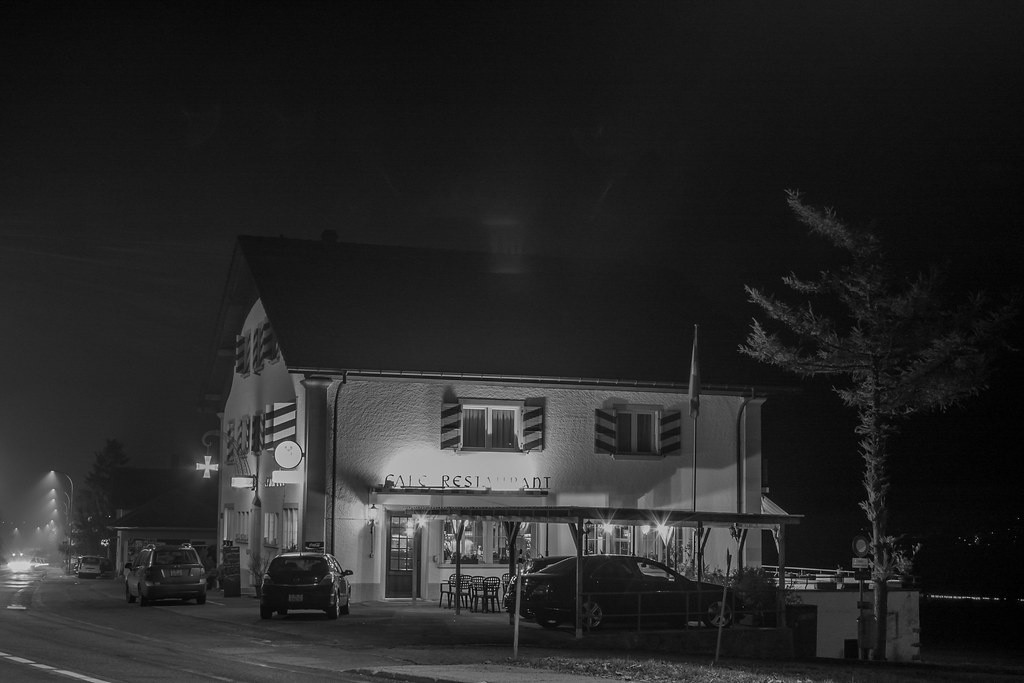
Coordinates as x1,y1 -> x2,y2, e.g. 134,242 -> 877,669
503,554 -> 742,633
259,551 -> 353,619
7,551 -> 49,571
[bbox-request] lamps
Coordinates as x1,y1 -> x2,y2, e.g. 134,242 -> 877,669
196,447 -> 219,478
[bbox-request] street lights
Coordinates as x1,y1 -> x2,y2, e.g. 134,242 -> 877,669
50,469 -> 73,574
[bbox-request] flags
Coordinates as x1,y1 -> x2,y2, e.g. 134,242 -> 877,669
689,324 -> 701,421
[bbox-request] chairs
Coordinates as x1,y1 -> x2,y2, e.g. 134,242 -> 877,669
439,573 -> 509,613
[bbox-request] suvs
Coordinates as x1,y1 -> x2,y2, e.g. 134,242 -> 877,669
124,541 -> 207,605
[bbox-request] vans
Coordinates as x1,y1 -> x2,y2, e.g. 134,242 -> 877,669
75,555 -> 101,579
